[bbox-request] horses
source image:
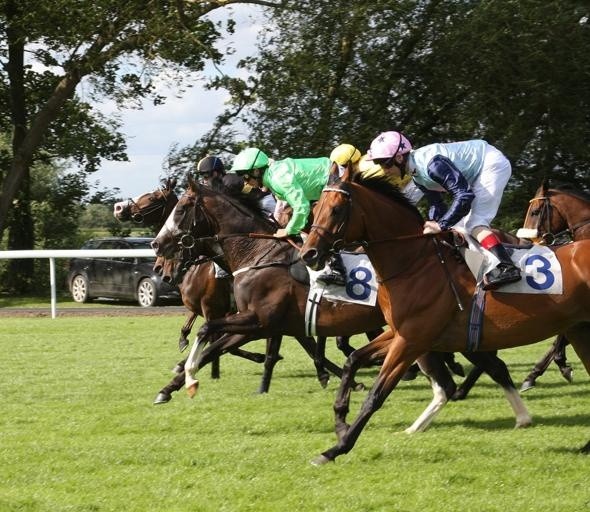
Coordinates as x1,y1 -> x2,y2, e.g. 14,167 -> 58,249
518,175 -> 589,393
152,170 -> 466,405
296,156 -> 588,465
113,178 -> 372,393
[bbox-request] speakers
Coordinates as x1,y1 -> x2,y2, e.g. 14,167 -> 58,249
318,250 -> 346,287
481,243 -> 520,289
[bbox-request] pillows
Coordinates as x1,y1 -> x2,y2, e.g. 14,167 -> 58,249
330,144 -> 361,166
365,132 -> 412,161
231,148 -> 268,171
193,158 -> 223,173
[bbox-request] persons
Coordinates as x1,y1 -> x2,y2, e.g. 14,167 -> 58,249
330,143 -> 425,207
230,148 -> 329,238
366,131 -> 522,291
195,156 -> 278,217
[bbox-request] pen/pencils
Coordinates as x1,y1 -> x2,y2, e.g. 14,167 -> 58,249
65,235 -> 182,308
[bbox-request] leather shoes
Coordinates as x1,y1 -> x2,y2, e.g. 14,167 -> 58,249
422,221 -> 442,234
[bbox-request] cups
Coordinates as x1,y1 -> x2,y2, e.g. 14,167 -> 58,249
203,158 -> 217,180
380,135 -> 402,168
243,150 -> 260,181
